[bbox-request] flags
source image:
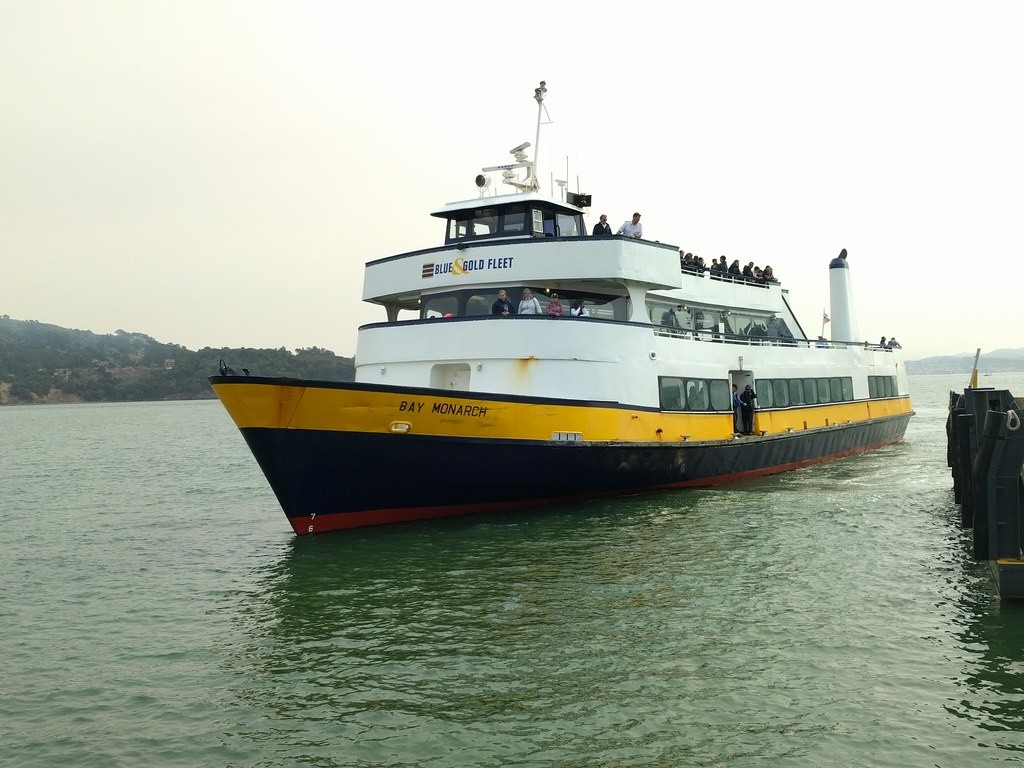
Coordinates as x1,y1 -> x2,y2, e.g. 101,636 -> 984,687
823,314 -> 830,325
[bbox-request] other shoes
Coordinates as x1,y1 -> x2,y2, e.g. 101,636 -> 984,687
749,432 -> 753,435
743,432 -> 747,435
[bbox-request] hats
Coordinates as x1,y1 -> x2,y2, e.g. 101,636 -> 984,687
551,293 -> 558,298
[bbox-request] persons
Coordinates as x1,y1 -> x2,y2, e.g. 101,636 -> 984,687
517,287 -> 543,315
678,249 -> 778,289
879,336 -> 886,349
731,384 -> 747,433
591,214 -> 612,235
570,298 -> 589,317
491,290 -> 515,316
888,337 -> 900,346
615,212 -> 642,238
544,292 -> 563,316
739,385 -> 757,435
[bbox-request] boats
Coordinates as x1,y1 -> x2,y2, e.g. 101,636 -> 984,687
204,78 -> 917,536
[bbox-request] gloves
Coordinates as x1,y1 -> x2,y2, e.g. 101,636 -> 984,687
743,403 -> 747,406
